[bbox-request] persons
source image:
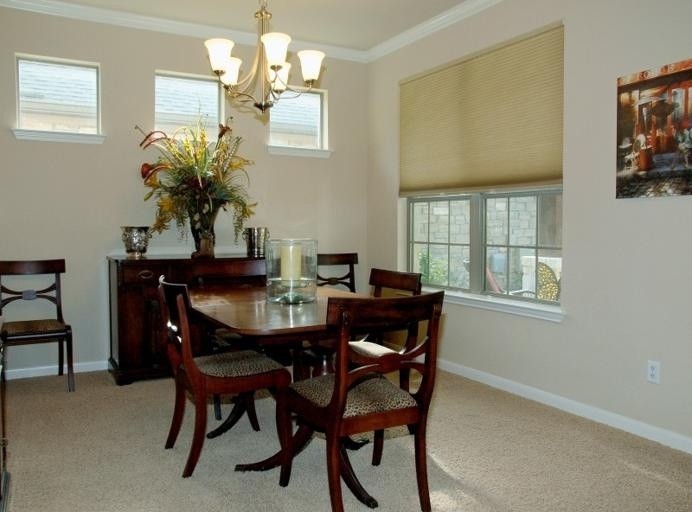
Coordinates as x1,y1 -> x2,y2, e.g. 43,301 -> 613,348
621,125 -> 692,171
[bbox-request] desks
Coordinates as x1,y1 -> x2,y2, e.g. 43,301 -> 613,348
104,256 -> 313,388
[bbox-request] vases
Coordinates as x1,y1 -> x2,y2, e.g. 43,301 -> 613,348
183,197 -> 224,258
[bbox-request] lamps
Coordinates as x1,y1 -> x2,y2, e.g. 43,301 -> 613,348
203,2 -> 326,120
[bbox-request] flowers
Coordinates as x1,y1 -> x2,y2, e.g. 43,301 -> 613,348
130,118 -> 264,244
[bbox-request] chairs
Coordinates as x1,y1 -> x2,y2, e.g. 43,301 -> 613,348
0,258 -> 80,393
153,252 -> 445,512
461,258 -> 535,299
